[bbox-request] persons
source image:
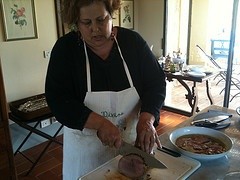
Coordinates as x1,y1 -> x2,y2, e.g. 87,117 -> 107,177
44,1 -> 167,180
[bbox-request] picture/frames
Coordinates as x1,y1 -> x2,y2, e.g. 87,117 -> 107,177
0,0 -> 38,42
54,0 -> 78,40
119,0 -> 134,30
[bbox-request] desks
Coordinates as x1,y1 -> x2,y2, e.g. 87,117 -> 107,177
161,70 -> 214,117
8,111 -> 63,177
77,104 -> 240,180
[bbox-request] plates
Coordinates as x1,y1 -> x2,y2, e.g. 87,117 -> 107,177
169,126 -> 233,160
236,105 -> 240,115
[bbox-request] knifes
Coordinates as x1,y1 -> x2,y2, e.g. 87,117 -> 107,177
118,142 -> 168,169
190,116 -> 229,125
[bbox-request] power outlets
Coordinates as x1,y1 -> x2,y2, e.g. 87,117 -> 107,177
41,118 -> 51,128
52,117 -> 58,124
44,51 -> 50,58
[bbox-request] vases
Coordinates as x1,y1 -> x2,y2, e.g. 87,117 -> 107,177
172,56 -> 184,68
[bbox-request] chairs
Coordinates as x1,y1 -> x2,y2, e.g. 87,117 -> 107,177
205,54 -> 240,102
196,44 -> 240,85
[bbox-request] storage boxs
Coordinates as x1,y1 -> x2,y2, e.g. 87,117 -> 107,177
10,93 -> 52,120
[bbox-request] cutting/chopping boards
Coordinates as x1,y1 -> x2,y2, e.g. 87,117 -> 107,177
78,142 -> 201,180
